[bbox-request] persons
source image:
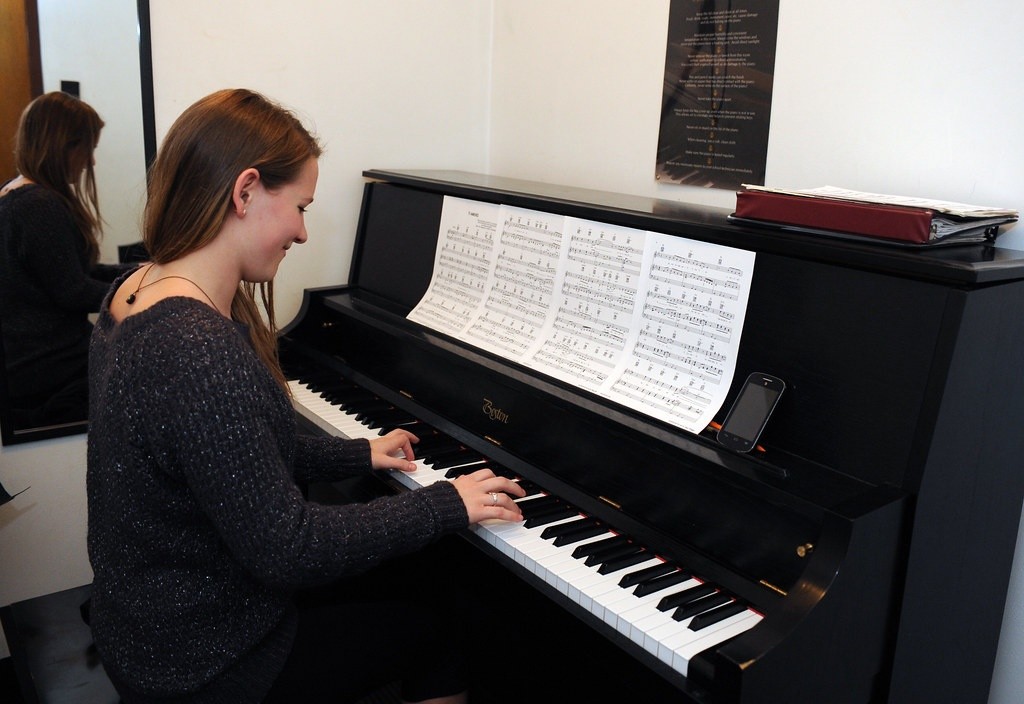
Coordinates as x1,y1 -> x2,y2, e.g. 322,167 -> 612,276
86,89 -> 527,704
0,91 -> 152,429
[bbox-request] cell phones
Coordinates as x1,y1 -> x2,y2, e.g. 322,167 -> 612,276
716,372 -> 786,452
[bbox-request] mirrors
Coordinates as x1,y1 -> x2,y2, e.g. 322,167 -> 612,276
0,0 -> 157,449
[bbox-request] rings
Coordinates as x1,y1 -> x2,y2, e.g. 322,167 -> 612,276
488,492 -> 497,507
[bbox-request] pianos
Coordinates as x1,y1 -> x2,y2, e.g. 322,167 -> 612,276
268,170 -> 1023,704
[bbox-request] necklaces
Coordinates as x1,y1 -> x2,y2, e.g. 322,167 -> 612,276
4,175 -> 26,192
125,262 -> 220,313
